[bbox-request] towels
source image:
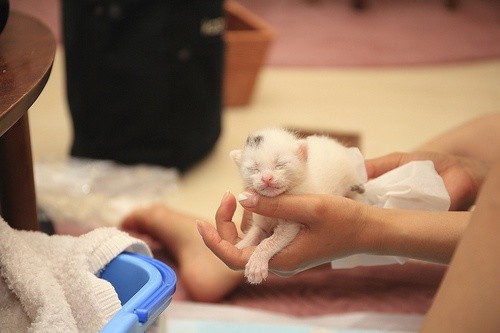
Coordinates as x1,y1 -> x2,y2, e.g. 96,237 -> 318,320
2,212 -> 155,329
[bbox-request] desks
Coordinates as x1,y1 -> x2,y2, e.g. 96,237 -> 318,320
0,8 -> 57,231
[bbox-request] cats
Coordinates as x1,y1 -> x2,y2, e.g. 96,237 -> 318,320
232,127 -> 369,285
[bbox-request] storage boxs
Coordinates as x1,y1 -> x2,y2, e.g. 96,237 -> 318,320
221,0 -> 274,106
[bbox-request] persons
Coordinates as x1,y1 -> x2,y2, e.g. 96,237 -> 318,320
119,113 -> 499,333
